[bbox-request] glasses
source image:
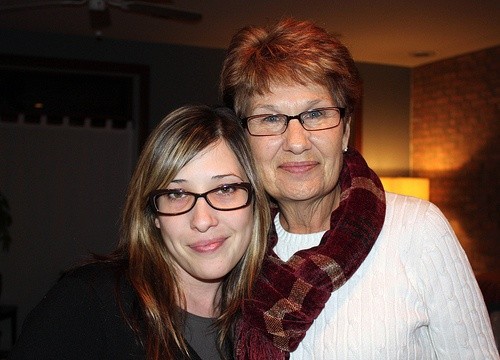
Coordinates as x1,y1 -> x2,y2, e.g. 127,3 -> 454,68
241,107 -> 348,137
149,182 -> 254,216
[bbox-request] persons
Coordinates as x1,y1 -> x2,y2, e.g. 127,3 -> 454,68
220,17 -> 498,360
7,105 -> 273,360
